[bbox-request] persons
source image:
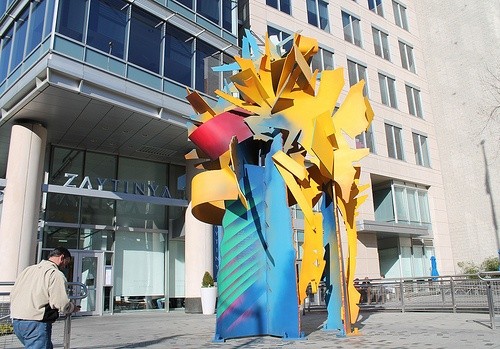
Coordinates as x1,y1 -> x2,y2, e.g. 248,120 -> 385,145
8,246 -> 82,349
361,276 -> 372,302
306,281 -> 314,302
354,278 -> 361,294
374,274 -> 391,302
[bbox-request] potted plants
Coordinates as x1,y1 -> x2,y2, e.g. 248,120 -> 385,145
200,272 -> 217,315
